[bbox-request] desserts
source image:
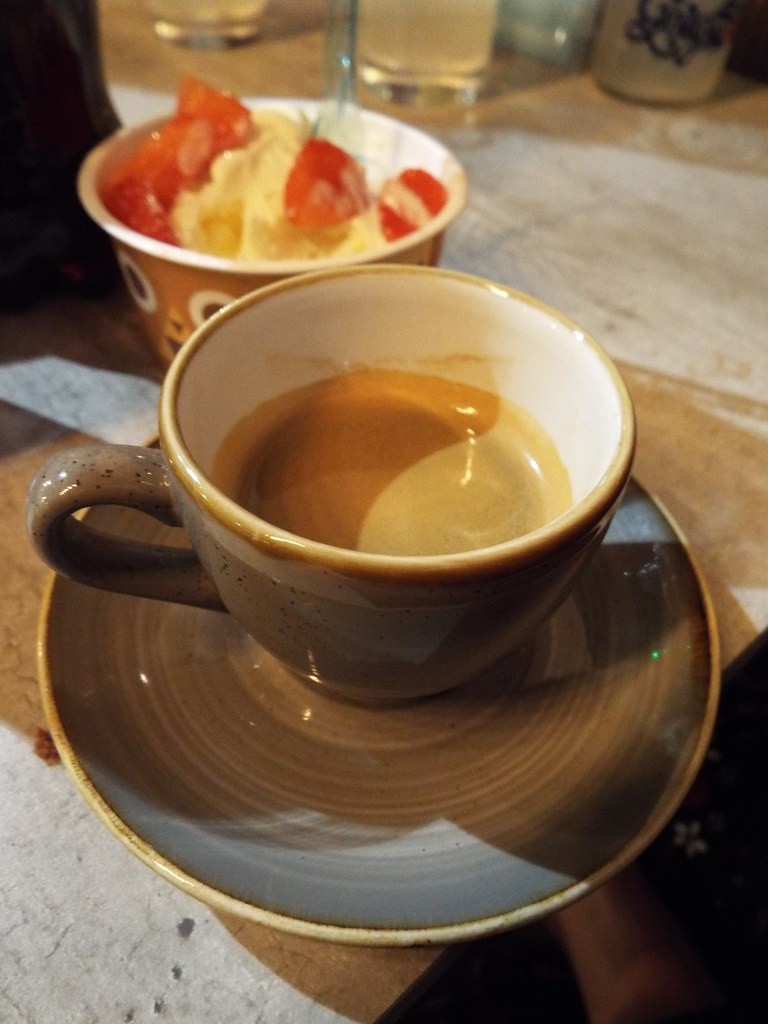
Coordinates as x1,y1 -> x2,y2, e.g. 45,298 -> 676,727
108,73 -> 454,263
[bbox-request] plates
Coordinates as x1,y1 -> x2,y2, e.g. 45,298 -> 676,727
35,430 -> 719,946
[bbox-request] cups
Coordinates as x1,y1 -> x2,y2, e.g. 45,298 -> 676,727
354,0 -> 499,110
23,264 -> 638,705
148,0 -> 269,47
593,0 -> 744,107
76,98 -> 471,365
498,0 -> 593,73
